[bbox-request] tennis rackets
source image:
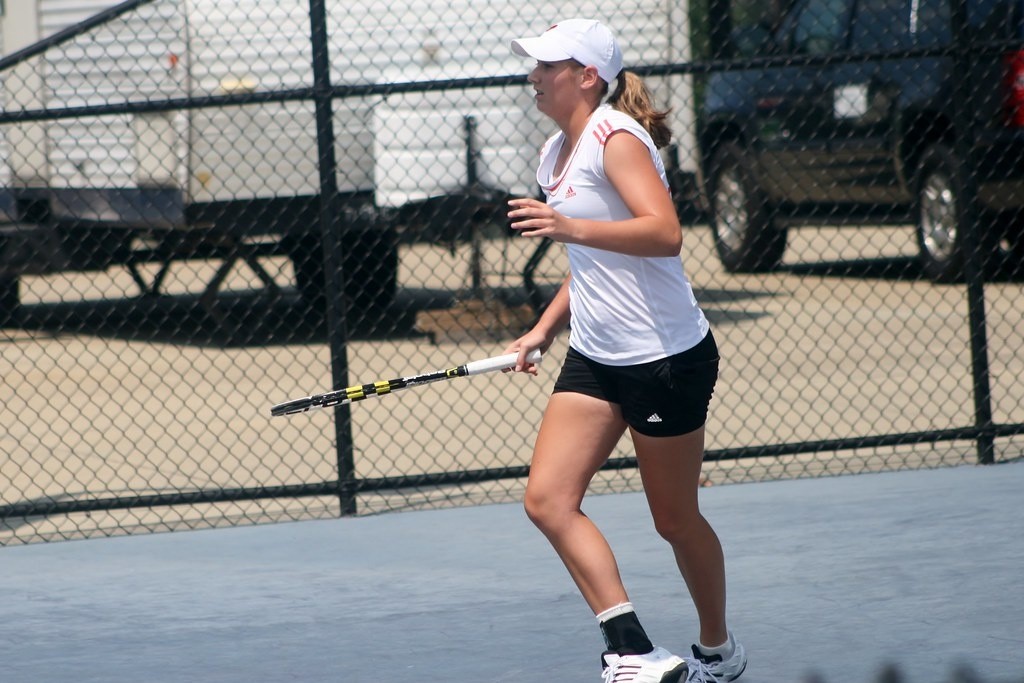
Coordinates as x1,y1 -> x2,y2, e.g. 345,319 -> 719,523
270,347 -> 544,418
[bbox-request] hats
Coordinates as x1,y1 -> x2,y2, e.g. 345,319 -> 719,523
510,18 -> 622,83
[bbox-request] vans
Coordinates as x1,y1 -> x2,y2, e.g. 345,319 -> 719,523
688,0 -> 1024,282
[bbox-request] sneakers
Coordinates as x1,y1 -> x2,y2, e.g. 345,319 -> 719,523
683,630 -> 747,683
600,645 -> 689,683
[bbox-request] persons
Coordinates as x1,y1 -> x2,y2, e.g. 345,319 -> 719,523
498,16 -> 747,683
